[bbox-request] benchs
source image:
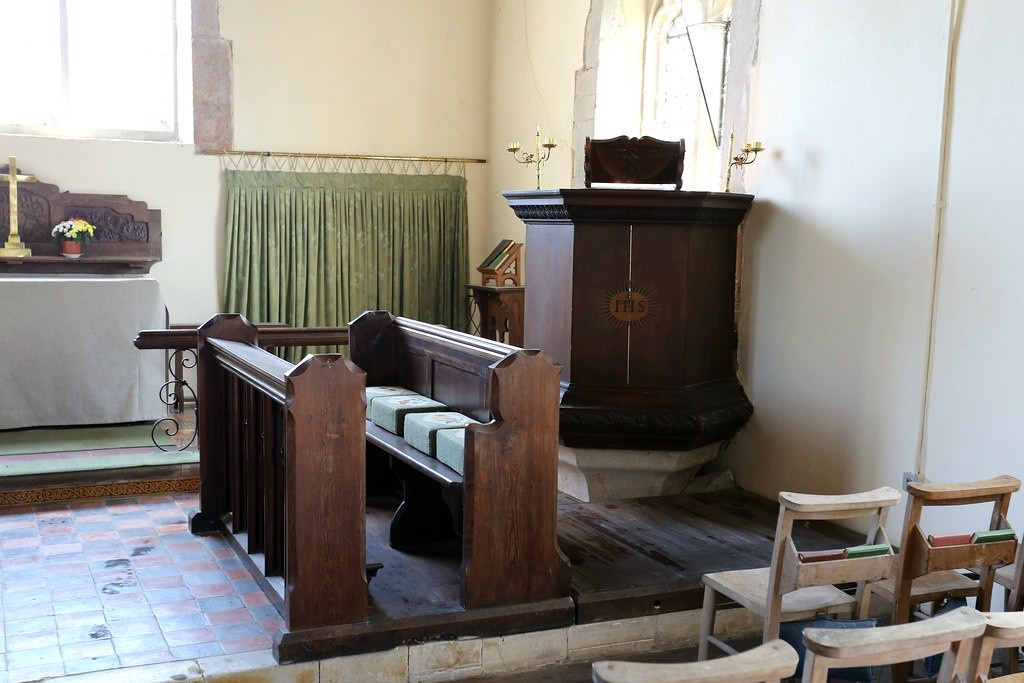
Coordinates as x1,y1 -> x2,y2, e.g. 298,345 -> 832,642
343,307 -> 572,611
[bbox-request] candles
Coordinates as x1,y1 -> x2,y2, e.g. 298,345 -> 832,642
544,137 -> 555,144
754,140 -> 761,148
508,140 -> 520,148
746,143 -> 752,148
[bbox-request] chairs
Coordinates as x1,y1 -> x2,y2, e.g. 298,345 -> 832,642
590,638 -> 805,683
861,471 -> 1022,628
695,483 -> 903,661
967,608 -> 1024,683
797,603 -> 988,683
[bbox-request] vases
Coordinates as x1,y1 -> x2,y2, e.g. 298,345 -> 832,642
62,238 -> 83,259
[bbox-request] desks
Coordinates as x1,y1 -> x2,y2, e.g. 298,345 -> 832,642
464,282 -> 525,349
501,188 -> 756,502
0,270 -> 168,433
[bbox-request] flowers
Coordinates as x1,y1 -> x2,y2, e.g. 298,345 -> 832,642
51,216 -> 97,245
